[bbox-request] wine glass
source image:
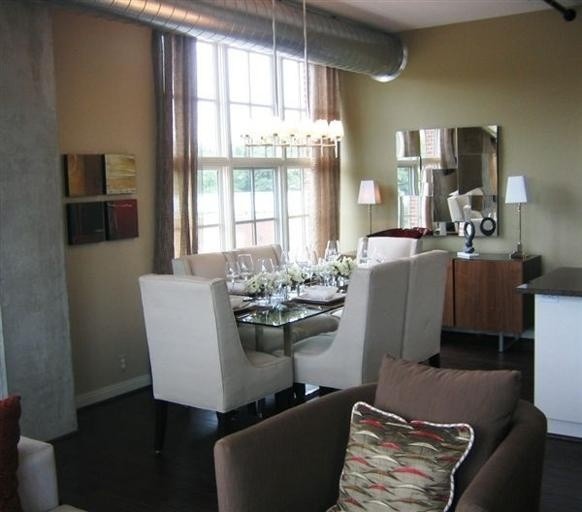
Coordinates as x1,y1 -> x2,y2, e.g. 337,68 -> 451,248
223,236 -> 386,321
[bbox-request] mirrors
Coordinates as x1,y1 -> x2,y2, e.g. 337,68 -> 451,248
461,221 -> 478,254
480,217 -> 495,235
395,125 -> 500,239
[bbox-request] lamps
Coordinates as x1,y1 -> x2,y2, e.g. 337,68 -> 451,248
358,181 -> 383,236
505,176 -> 530,259
237,0 -> 344,156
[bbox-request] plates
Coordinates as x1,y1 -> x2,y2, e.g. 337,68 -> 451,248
292,291 -> 347,306
232,302 -> 256,314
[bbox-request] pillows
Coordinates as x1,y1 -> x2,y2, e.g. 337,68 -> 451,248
374,351 -> 520,480
320,402 -> 473,512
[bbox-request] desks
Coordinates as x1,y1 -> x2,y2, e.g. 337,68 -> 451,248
438,250 -> 538,351
514,266 -> 582,440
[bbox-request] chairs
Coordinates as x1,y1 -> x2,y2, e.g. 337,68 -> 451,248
213,379 -> 546,512
0,396 -> 61,512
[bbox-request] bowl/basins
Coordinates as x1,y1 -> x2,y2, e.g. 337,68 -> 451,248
304,285 -> 338,301
228,296 -> 243,308
